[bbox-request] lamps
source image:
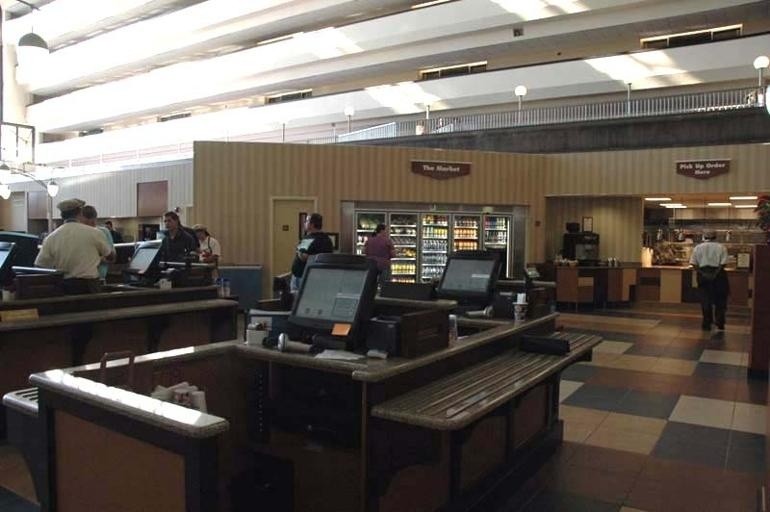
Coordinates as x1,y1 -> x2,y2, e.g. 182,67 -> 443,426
753,55 -> 770,94
623,75 -> 635,116
47,167 -> 64,198
344,107 -> 355,132
424,97 -> 433,119
514,85 -> 528,125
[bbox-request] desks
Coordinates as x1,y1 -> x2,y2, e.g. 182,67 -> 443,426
543,265 -> 641,313
642,266 -> 694,304
724,267 -> 749,307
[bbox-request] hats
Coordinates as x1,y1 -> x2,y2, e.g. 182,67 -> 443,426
703,232 -> 715,238
191,224 -> 208,231
56,198 -> 85,211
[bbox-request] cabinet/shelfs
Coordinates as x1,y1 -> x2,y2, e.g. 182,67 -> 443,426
747,244 -> 770,381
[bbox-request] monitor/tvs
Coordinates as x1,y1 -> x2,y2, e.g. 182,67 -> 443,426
434,250 -> 507,300
0,242 -> 17,279
125,241 -> 164,279
287,252 -> 385,336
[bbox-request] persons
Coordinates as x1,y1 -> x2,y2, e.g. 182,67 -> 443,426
79,206 -> 117,279
362,224 -> 396,282
35,199 -> 116,293
690,229 -> 729,330
104,221 -> 124,244
192,225 -> 220,267
163,212 -> 197,264
290,213 -> 333,293
